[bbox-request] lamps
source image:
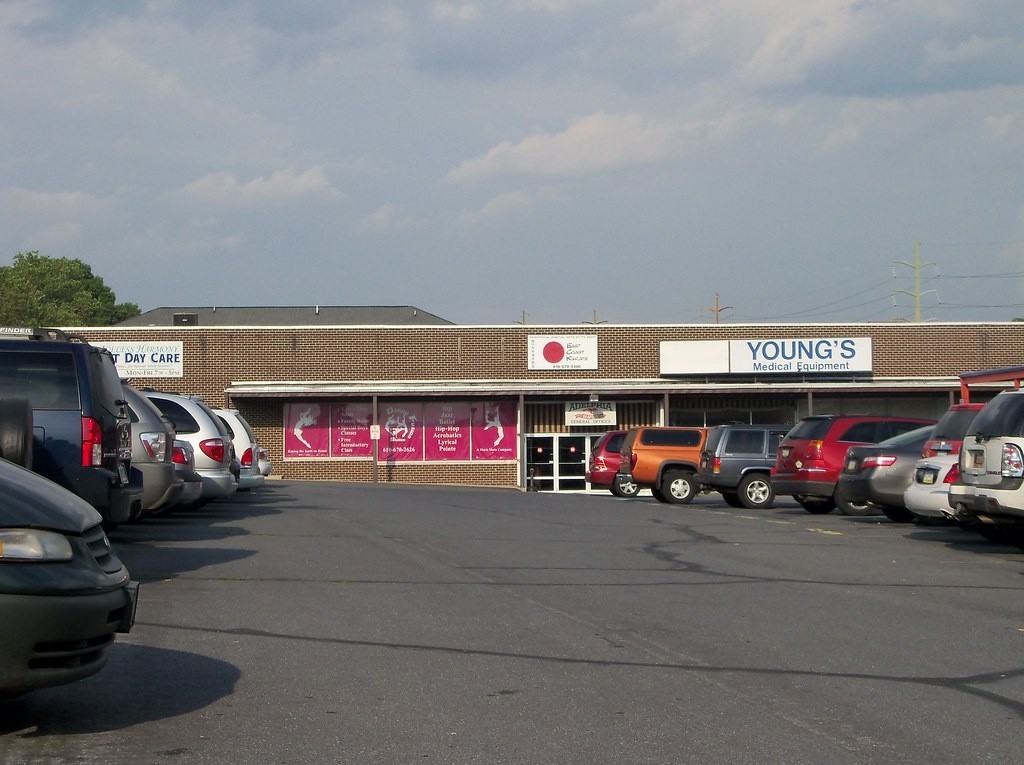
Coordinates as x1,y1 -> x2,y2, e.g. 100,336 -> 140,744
589,395 -> 599,403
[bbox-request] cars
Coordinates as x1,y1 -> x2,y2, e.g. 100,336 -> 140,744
903,402 -> 989,535
585,430 -> 650,498
838,423 -> 940,523
257,450 -> 272,478
0,457 -> 140,694
616,426 -> 712,505
117,375 -> 187,524
149,440 -> 204,516
946,387 -> 1024,545
770,413 -> 940,516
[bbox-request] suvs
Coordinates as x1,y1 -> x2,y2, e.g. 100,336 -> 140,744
0,325 -> 144,525
137,386 -> 241,501
690,424 -> 798,510
208,405 -> 265,490
